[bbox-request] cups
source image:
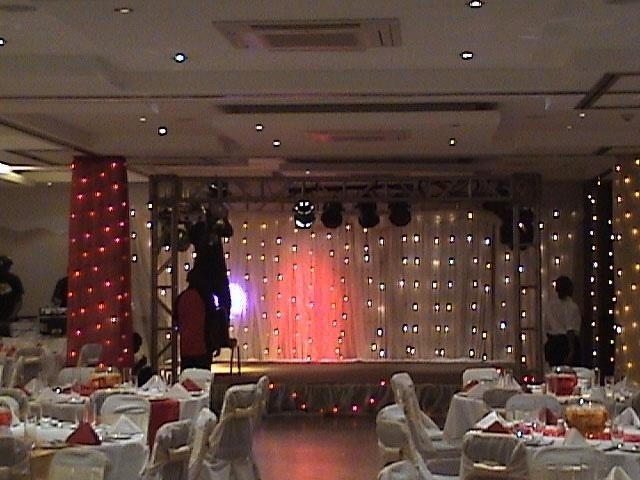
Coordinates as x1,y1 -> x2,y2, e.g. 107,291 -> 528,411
123,367 -> 139,395
534,421 -> 546,444
590,369 -> 601,390
75,403 -> 96,425
604,376 -> 615,401
71,385 -> 81,402
23,412 -> 37,450
40,397 -> 58,428
32,372 -> 48,401
504,368 -> 514,390
610,413 -> 625,447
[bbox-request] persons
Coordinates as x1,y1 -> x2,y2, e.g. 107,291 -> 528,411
173,269 -> 221,376
543,274 -> 584,369
134,334 -> 157,387
0,256 -> 24,336
184,198 -> 238,346
51,266 -> 68,305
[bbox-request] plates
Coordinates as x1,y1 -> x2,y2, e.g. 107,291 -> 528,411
39,442 -> 69,449
619,444 -> 640,452
112,435 -> 131,439
522,438 -> 555,446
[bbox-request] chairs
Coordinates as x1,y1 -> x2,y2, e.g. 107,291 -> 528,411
379,366 -> 640,479
0,343 -> 270,480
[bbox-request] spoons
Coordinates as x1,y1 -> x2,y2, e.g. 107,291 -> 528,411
631,445 -> 639,451
602,443 -> 623,451
